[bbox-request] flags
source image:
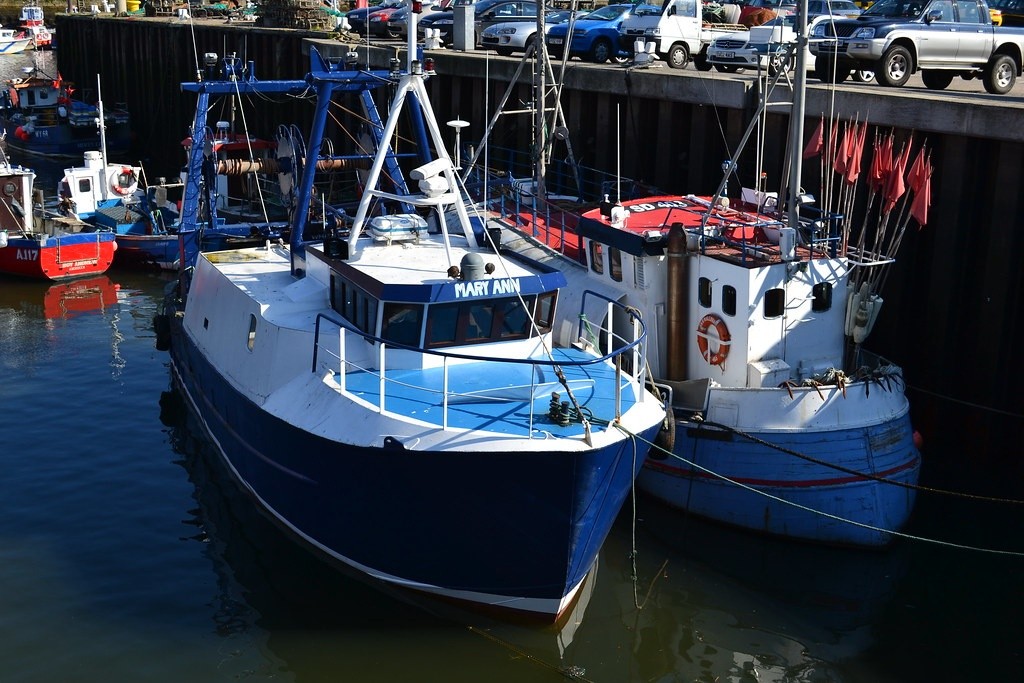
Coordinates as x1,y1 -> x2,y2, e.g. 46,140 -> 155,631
907,145 -> 924,192
910,176 -> 928,226
835,119 -> 911,215
915,158 -> 931,211
803,120 -> 823,160
823,118 -> 838,164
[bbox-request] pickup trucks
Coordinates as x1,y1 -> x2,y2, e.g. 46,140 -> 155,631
807,0 -> 1023,95
749,0 -> 802,18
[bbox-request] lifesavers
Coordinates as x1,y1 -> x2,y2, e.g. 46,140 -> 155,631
37,34 -> 42,40
43,32 -> 49,39
109,167 -> 138,194
696,314 -> 729,365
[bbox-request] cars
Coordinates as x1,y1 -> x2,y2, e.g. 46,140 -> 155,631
386,0 -> 454,43
480,9 -> 593,59
808,0 -> 863,19
417,0 -> 567,48
704,13 -> 876,83
343,1 -> 404,37
363,1 -> 411,38
545,3 -> 664,64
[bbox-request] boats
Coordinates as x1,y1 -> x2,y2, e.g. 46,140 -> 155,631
155,115 -> 342,253
43,74 -> 190,274
0,159 -> 119,283
452,0 -> 925,545
1,34 -> 129,167
170,0 -> 672,627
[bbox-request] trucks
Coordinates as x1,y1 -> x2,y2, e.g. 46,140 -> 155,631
618,1 -> 753,76
